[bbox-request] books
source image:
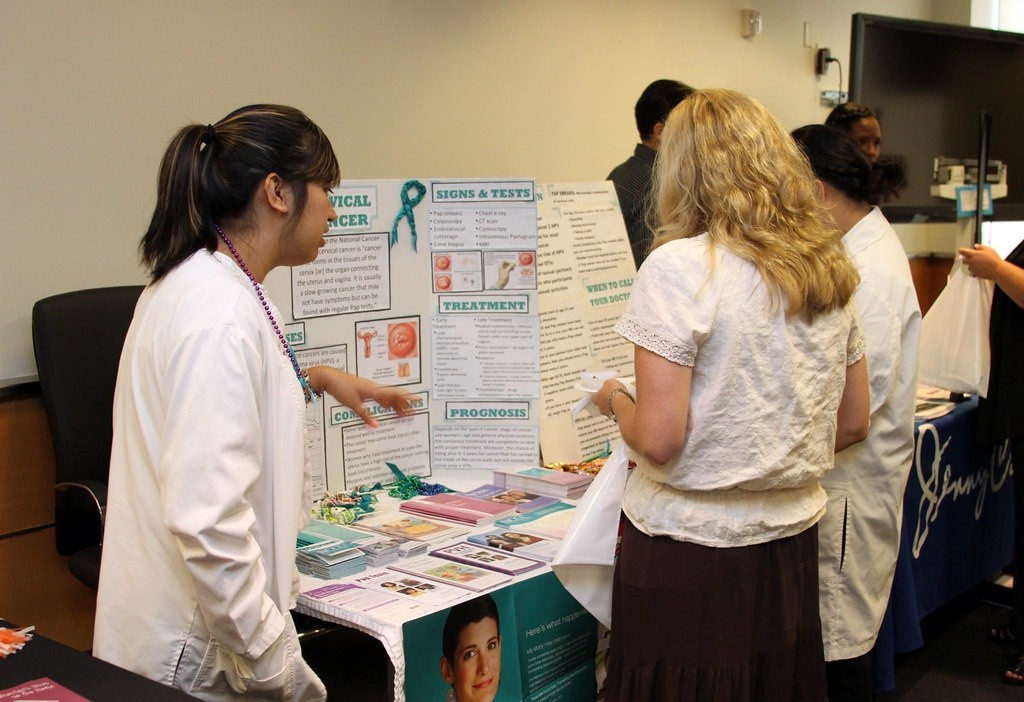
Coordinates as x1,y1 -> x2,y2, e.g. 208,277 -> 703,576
289,461 -> 605,630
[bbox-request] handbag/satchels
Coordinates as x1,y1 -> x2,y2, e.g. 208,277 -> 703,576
918,255 -> 992,400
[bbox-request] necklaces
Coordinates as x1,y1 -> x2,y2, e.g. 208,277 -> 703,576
215,223 -> 312,409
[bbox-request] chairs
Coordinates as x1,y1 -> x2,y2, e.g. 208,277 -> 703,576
28,284 -> 148,591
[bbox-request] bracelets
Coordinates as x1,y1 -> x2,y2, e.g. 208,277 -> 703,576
301,368 -> 321,401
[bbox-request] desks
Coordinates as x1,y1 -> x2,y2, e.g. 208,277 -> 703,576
290,389 -> 1009,699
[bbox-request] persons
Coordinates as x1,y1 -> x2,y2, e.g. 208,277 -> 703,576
785,124 -> 924,702
93,104 -> 415,700
957,235 -> 1024,685
600,77 -> 705,271
820,103 -> 884,173
593,88 -> 871,702
441,594 -> 503,702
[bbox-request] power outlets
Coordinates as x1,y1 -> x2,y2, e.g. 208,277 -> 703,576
817,47 -> 833,75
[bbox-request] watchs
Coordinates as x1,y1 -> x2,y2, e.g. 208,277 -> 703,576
607,388 -> 636,423
